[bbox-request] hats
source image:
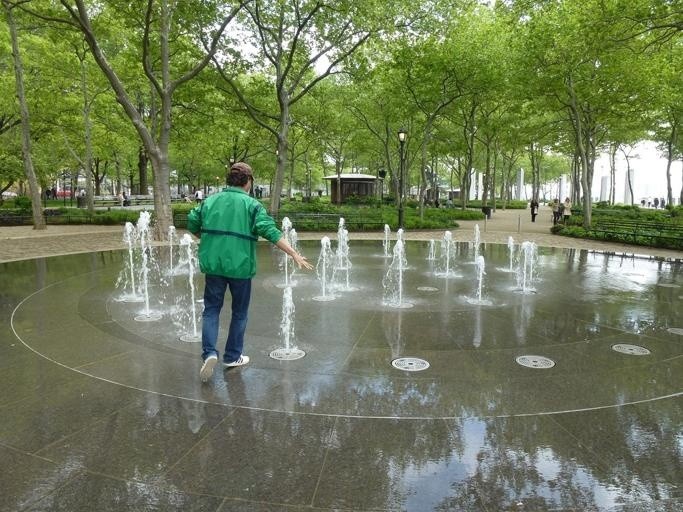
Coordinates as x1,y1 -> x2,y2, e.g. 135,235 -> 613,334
230,162 -> 254,181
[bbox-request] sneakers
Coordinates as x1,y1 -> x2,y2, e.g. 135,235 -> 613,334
200,355 -> 217,382
223,355 -> 249,366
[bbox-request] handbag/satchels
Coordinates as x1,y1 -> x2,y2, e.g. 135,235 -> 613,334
534,208 -> 537,215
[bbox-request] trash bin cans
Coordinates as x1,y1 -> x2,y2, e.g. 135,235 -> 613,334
482,207 -> 490,219
76,196 -> 85,208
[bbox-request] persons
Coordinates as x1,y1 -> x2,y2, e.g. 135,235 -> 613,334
529,196 -> 539,222
640,196 -> 666,210
185,162 -> 316,383
319,190 -> 322,198
435,199 -> 440,208
162,365 -> 290,512
447,197 -> 455,208
43,184 -> 264,210
551,196 -> 572,226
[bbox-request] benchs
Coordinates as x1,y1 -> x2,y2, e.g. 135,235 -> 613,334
584,218 -> 682,251
92,198 -> 181,208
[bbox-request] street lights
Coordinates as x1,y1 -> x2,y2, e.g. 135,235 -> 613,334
216,177 -> 220,193
225,165 -> 227,188
305,168 -> 312,196
398,130 -> 407,233
